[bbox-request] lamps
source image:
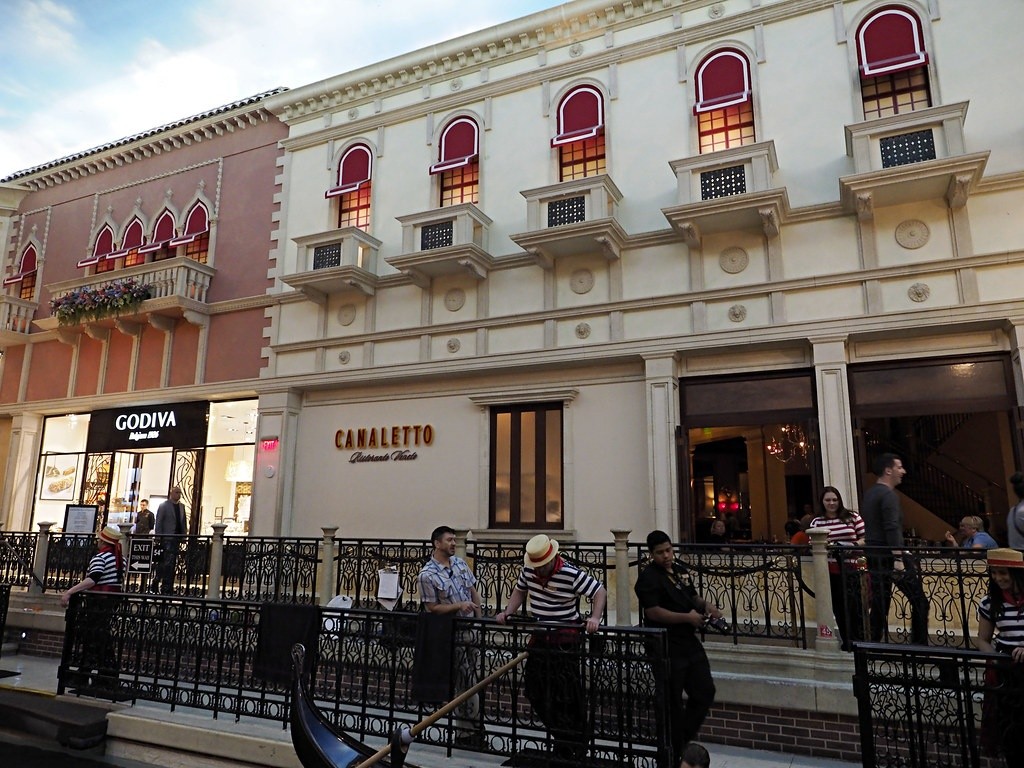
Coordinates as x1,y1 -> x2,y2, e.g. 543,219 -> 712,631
767,422 -> 809,470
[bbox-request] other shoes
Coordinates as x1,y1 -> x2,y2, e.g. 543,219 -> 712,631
161,589 -> 171,596
151,580 -> 158,592
454,735 -> 488,748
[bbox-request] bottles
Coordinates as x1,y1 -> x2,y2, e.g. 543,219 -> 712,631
758,535 -> 786,552
903,527 -> 942,554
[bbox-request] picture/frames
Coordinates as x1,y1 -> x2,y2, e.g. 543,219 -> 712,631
39,451 -> 79,501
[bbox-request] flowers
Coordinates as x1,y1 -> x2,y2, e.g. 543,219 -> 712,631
47,278 -> 154,329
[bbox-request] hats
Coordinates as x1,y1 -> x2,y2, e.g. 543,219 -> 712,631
524,533 -> 558,568
98,524 -> 122,545
982,548 -> 1024,568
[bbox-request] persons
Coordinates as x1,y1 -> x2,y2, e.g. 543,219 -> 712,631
1007,472 -> 1024,550
977,548 -> 1024,768
60,525 -> 122,677
417,526 -> 490,748
152,487 -> 187,586
496,535 -> 607,757
809,486 -> 866,651
862,454 -> 929,645
677,743 -> 710,768
634,530 -> 723,742
785,514 -> 814,556
706,520 -> 736,551
133,499 -> 155,578
944,515 -> 999,555
